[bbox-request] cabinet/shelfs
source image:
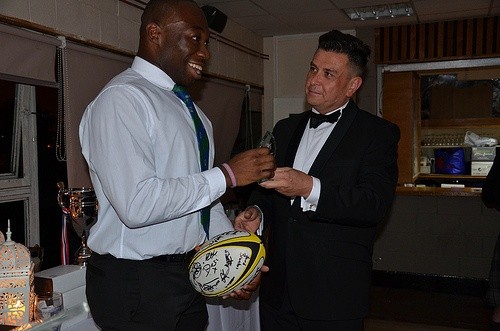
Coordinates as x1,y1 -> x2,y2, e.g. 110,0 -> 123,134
420,145 -> 500,177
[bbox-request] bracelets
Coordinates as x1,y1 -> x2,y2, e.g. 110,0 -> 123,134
222,163 -> 237,187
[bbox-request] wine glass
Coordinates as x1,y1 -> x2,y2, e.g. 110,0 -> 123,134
421,133 -> 464,146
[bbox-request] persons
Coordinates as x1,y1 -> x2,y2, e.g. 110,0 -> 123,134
78,0 -> 276,331
234,28 -> 401,331
480,151 -> 500,307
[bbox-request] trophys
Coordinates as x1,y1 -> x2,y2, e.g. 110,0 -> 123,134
58,183 -> 99,267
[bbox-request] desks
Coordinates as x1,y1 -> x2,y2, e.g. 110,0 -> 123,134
29,264 -> 260,331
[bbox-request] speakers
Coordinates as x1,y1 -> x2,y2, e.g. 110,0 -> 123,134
201,5 -> 228,33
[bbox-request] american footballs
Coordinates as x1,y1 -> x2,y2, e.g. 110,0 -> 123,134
187,230 -> 266,299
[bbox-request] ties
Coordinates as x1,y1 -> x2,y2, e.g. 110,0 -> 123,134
173,84 -> 210,240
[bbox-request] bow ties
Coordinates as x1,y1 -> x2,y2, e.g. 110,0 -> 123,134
309,109 -> 341,130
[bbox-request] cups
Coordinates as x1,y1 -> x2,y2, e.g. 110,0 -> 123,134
33,292 -> 63,331
